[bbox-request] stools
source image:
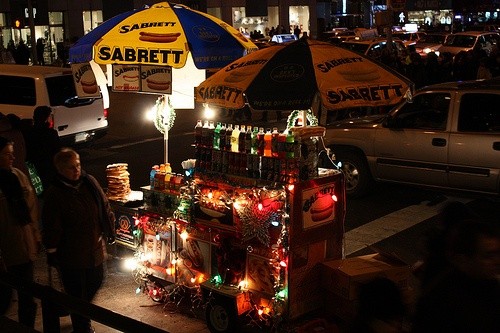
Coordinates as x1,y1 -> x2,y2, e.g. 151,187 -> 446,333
320,252 -> 416,323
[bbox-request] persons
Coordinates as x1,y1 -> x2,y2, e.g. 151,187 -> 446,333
40,149 -> 119,333
26,105 -> 60,170
0,135 -> 45,332
248,11 -> 499,81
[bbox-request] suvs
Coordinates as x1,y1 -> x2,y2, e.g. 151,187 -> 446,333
0,64 -> 110,144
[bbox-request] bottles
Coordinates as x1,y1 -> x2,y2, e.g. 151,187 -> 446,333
150,163 -> 185,192
194,119 -> 300,182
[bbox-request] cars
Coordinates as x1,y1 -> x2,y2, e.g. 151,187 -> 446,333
319,28 -> 499,73
318,80 -> 499,209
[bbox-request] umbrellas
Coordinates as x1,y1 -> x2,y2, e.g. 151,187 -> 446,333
193,28 -> 413,125
69,1 -> 259,170
